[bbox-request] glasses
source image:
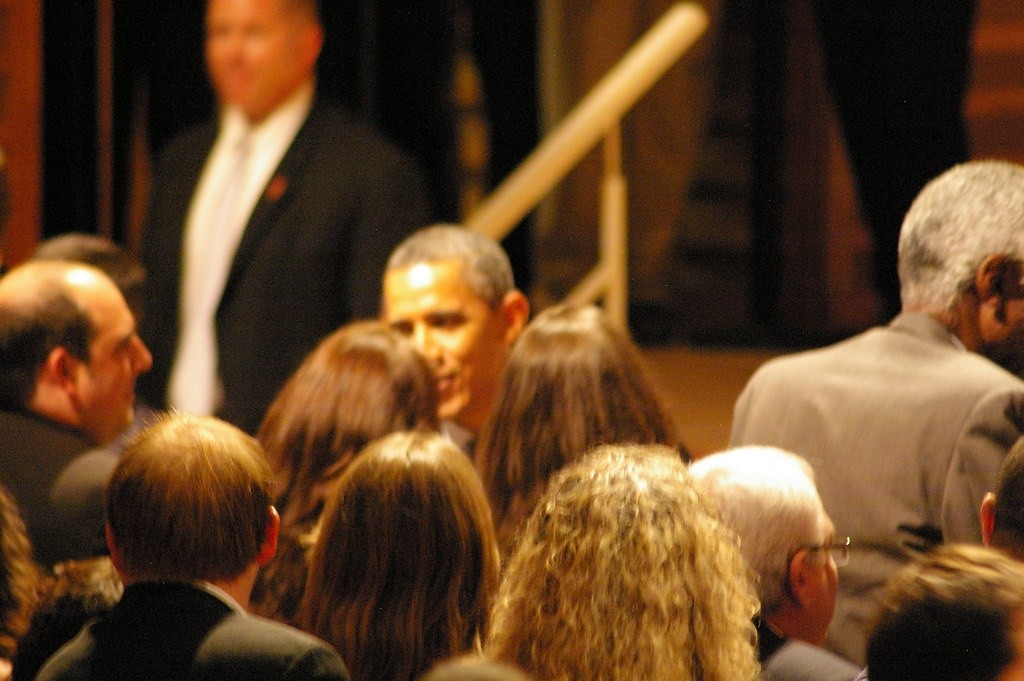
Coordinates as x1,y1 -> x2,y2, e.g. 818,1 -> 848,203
791,535 -> 852,568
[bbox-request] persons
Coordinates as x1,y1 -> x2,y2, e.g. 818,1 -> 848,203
138,1 -> 430,437
1,157 -> 1024,681
